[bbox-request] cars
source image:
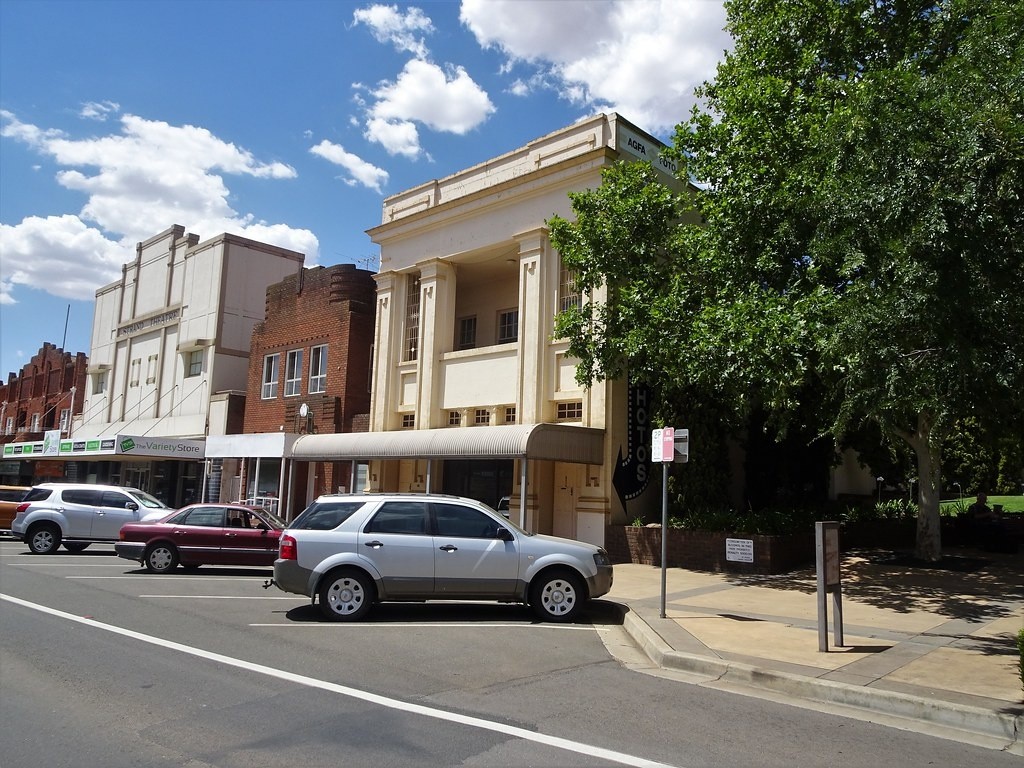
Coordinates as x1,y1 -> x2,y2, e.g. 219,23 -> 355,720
114,502 -> 291,573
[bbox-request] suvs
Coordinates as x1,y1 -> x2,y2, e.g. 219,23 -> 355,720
12,483 -> 180,556
0,484 -> 32,539
263,493 -> 613,620
